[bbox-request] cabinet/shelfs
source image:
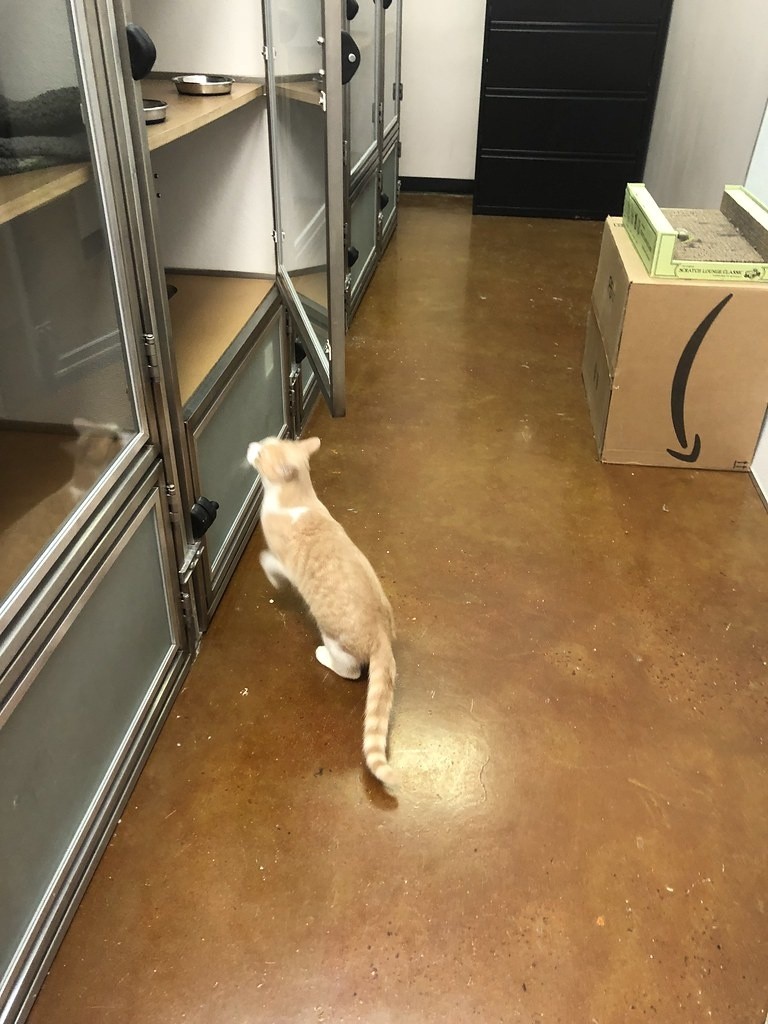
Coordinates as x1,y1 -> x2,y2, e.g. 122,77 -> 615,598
0,0 -> 198,1023
472,1 -> 674,222
82,0 -> 405,635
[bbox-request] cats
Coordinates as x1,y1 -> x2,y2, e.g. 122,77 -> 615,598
247,436 -> 396,789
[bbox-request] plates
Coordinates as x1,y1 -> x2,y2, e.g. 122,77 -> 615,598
142,98 -> 168,121
171,74 -> 235,95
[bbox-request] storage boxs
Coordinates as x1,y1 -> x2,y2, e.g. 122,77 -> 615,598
580,215 -> 768,472
